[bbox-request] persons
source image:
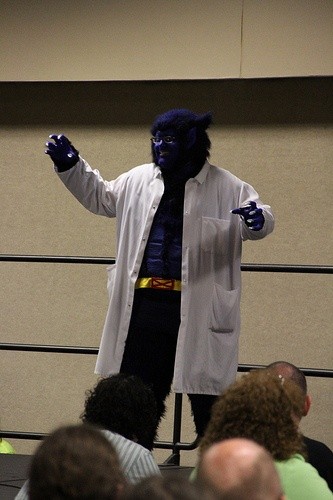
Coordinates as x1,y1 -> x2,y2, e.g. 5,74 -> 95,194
196,438 -> 286,500
14,374 -> 168,500
190,369 -> 333,500
266,360 -> 333,494
28,426 -> 130,500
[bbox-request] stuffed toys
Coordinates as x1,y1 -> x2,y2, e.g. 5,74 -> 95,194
46,109 -> 274,440
